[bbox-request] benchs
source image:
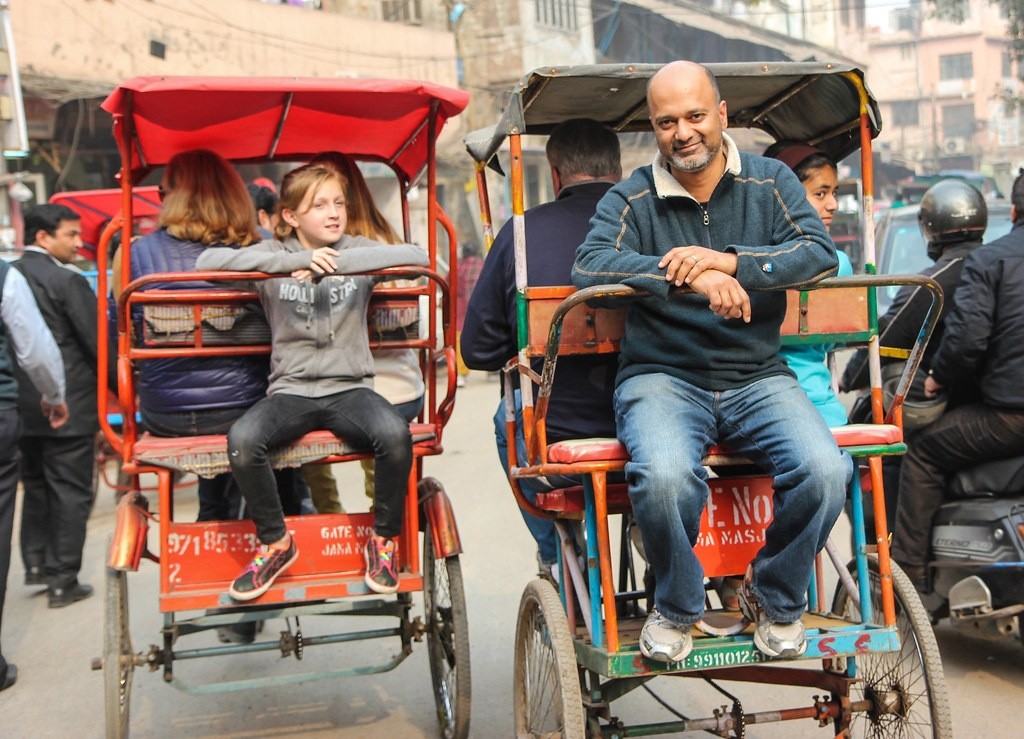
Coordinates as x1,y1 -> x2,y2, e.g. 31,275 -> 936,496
118,289 -> 448,471
524,276 -> 910,508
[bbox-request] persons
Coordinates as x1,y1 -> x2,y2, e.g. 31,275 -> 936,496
839,167 -> 1024,598
459,117 -> 624,594
571,61 -> 839,665
104,149 -> 426,649
0,256 -> 68,692
761,140 -> 855,460
443,241 -> 488,389
194,164 -> 432,601
6,204 -> 104,609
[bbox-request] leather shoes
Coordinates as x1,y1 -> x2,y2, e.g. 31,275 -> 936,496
25,566 -> 93,608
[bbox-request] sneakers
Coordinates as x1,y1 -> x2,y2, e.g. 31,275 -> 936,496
229,530 -> 299,601
487,374 -> 500,384
736,564 -> 807,660
457,375 -> 468,387
364,534 -> 401,593
639,597 -> 694,665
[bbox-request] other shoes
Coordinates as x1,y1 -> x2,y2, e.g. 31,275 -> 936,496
208,607 -> 256,643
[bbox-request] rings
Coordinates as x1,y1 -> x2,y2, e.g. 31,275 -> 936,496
690,254 -> 698,264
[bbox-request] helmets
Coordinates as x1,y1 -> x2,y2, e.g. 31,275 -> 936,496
916,178 -> 987,243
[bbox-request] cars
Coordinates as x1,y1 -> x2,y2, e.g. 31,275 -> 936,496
872,201 -> 1021,344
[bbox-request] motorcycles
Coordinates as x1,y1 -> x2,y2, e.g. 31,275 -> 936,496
840,345 -> 1024,656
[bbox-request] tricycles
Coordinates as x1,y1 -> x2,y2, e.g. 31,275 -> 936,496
93,76 -> 476,737
462,60 -> 959,737
